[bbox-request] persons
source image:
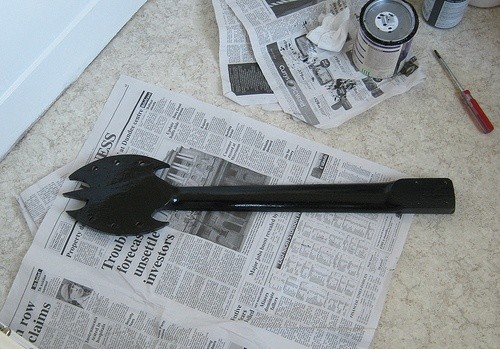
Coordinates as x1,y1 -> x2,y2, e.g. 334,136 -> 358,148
60,282 -> 93,309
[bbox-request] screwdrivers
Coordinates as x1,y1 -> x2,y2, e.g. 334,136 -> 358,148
434,49 -> 494,134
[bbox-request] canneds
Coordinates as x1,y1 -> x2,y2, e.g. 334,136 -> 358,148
351,1 -> 419,80
422,0 -> 470,30
